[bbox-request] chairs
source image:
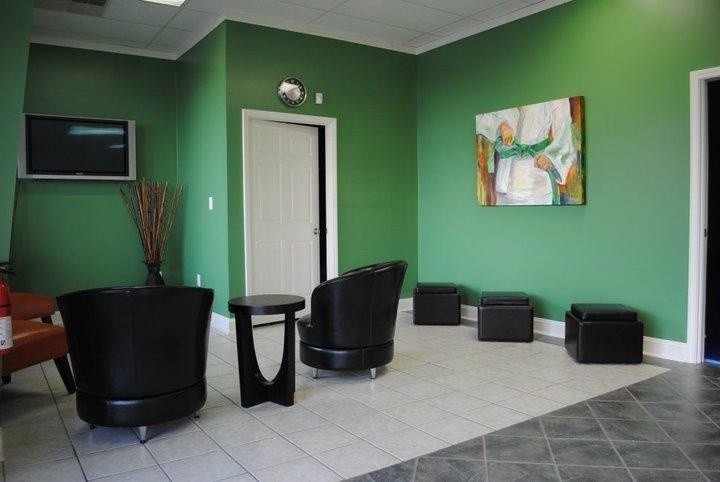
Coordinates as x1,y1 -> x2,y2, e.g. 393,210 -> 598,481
293,259 -> 410,380
48,284 -> 215,445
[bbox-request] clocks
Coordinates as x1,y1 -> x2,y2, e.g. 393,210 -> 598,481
276,77 -> 307,108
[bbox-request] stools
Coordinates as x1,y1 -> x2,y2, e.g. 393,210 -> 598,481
0,290 -> 75,398
414,278 -> 644,365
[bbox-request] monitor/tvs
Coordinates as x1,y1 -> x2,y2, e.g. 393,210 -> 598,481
18,112 -> 137,184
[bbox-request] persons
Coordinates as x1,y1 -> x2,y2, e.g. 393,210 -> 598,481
475,98 -> 579,207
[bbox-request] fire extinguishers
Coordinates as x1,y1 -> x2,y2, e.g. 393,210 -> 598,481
0,260 -> 17,355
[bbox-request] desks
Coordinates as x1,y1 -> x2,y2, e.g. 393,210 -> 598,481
227,294 -> 306,407
